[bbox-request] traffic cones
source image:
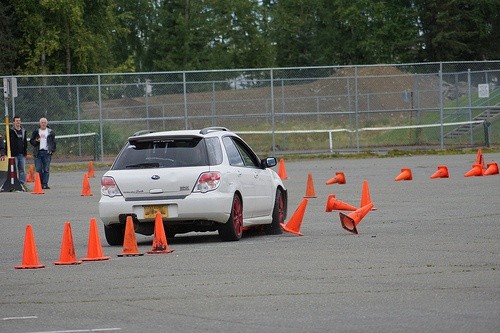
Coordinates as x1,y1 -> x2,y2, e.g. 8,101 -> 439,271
324,194 -> 356,212
464,164 -> 484,177
279,198 -> 309,236
476,148 -> 485,167
325,171 -> 346,185
483,162 -> 500,176
358,179 -> 378,210
51,220 -> 81,265
26,164 -> 35,182
31,173 -> 45,194
80,217 -> 111,261
278,156 -> 287,179
430,166 -> 449,178
14,223 -> 47,270
304,172 -> 318,199
393,168 -> 414,181
80,173 -> 94,196
338,203 -> 374,237
145,213 -> 177,255
115,215 -> 146,256
87,160 -> 96,178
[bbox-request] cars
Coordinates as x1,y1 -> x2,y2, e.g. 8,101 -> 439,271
98,124 -> 288,243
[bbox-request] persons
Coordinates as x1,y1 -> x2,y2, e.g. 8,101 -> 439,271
4,115 -> 27,185
29,117 -> 56,189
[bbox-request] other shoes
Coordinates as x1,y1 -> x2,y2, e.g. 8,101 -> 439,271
42,185 -> 50,189
